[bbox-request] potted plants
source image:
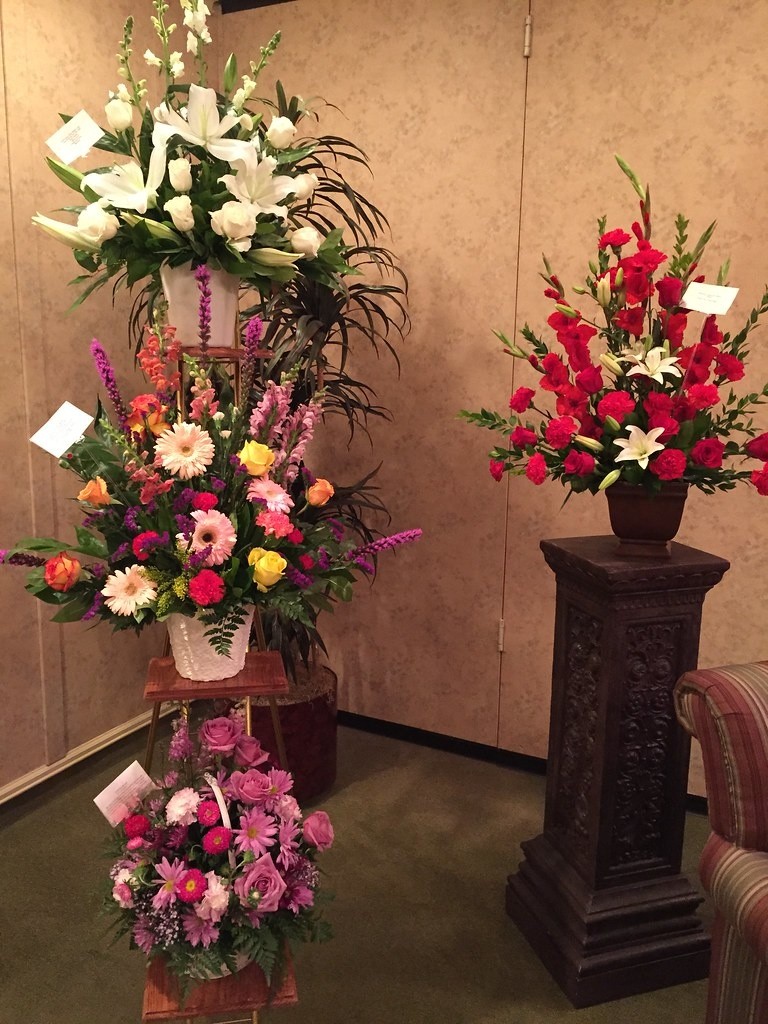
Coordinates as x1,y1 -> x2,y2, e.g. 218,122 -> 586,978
107,267 -> 401,806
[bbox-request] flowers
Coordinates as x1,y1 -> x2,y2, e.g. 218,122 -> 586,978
0,266 -> 422,687
450,153 -> 768,497
99,703 -> 335,1016
29,0 -> 414,385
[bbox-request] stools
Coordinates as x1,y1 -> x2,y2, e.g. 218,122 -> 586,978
144,651 -> 290,782
141,939 -> 298,1024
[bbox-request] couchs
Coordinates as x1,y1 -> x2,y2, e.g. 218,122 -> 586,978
672,662 -> 768,1024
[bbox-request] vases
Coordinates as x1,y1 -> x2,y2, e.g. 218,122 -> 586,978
605,482 -> 689,557
164,604 -> 256,682
160,255 -> 237,349
171,946 -> 255,980
